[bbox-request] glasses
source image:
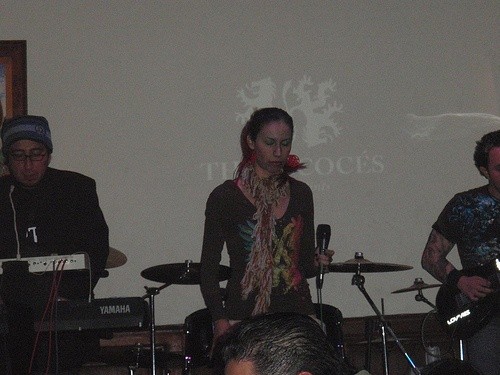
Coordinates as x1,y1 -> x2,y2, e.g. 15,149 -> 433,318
8,151 -> 48,162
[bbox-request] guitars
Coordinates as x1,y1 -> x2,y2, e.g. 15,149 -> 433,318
436,256 -> 500,338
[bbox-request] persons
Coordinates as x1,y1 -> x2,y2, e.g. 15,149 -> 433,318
420,130 -> 500,375
0,114 -> 109,375
197,106 -> 333,348
211,312 -> 369,375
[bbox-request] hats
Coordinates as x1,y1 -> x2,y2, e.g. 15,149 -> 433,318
0,115 -> 53,157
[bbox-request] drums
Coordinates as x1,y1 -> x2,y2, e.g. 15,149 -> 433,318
316,303 -> 345,360
181,308 -> 222,364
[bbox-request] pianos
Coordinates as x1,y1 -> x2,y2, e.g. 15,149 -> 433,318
0,253 -> 151,375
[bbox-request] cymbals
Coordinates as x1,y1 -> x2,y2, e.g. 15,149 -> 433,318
328,258 -> 413,275
393,281 -> 441,294
139,260 -> 230,285
105,246 -> 129,271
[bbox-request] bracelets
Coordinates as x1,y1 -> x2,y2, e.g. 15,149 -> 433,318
446,269 -> 462,287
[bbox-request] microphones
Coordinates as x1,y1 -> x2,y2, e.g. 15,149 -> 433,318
316,224 -> 331,288
9,185 -> 21,259
